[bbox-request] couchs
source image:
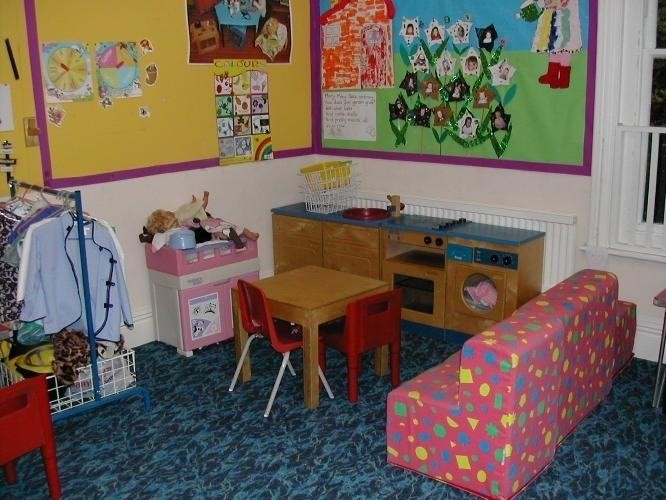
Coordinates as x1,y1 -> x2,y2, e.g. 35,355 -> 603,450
385,269 -> 637,500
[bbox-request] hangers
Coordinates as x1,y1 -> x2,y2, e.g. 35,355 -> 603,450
1,179 -> 90,237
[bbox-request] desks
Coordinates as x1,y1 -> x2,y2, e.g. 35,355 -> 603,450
230,263 -> 391,409
652,288 -> 666,408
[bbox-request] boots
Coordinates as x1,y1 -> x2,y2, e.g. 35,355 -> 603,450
550,65 -> 572,89
537,61 -> 561,84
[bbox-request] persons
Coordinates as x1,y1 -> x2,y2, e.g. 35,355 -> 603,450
531,0 -> 582,88
253,0 -> 267,18
394,23 -> 509,135
146,191 -> 209,235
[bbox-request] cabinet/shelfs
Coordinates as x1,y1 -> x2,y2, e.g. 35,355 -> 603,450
270,212 -> 546,346
144,237 -> 261,357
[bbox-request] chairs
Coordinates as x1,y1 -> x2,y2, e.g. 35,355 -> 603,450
0,373 -> 64,500
320,288 -> 405,404
236,276 -> 329,390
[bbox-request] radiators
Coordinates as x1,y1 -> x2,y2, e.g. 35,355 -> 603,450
343,195 -> 578,293
